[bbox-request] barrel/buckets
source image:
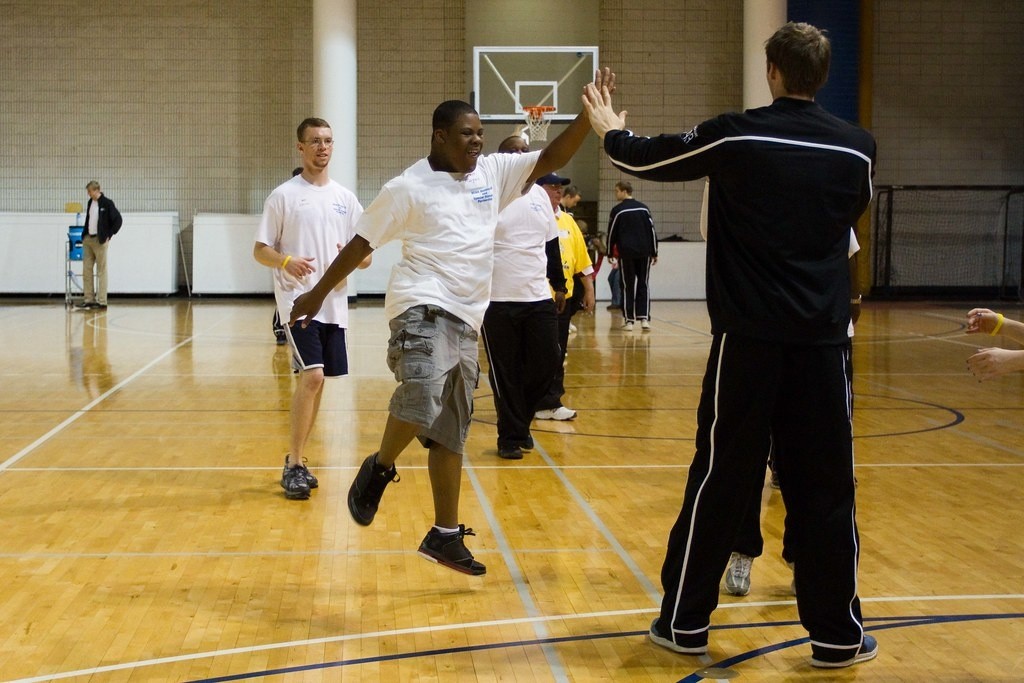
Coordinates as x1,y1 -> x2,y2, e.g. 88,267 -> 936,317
67,226 -> 86,261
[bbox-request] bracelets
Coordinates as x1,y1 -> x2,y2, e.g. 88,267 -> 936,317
281,256 -> 291,271
990,313 -> 1004,337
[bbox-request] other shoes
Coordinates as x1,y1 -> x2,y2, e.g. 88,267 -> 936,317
620,321 -> 633,330
567,322 -> 577,334
640,319 -> 651,327
73,302 -> 106,311
277,329 -> 288,344
606,303 -> 622,311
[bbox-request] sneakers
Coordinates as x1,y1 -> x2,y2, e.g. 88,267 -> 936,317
282,454 -> 319,490
519,427 -> 534,451
349,451 -> 399,528
278,465 -> 310,500
648,617 -> 709,654
810,634 -> 877,667
534,402 -> 577,420
785,559 -> 797,596
497,443 -> 524,459
725,551 -> 754,595
415,524 -> 487,576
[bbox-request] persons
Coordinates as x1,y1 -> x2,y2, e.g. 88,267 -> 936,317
480,135 -> 568,460
272,167 -> 304,346
699,177 -> 862,600
559,180 -> 658,335
290,64 -> 616,576
254,117 -> 372,499
535,171 -> 595,366
74,180 -> 123,311
582,22 -> 878,668
963,308 -> 1024,383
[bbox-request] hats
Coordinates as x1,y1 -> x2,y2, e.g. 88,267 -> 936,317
536,170 -> 572,188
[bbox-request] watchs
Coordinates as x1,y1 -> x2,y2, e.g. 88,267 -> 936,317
850,294 -> 862,305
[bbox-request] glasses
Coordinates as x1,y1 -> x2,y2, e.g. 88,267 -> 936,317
298,138 -> 334,148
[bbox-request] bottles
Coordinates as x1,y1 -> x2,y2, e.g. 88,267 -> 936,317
76,212 -> 81,226
